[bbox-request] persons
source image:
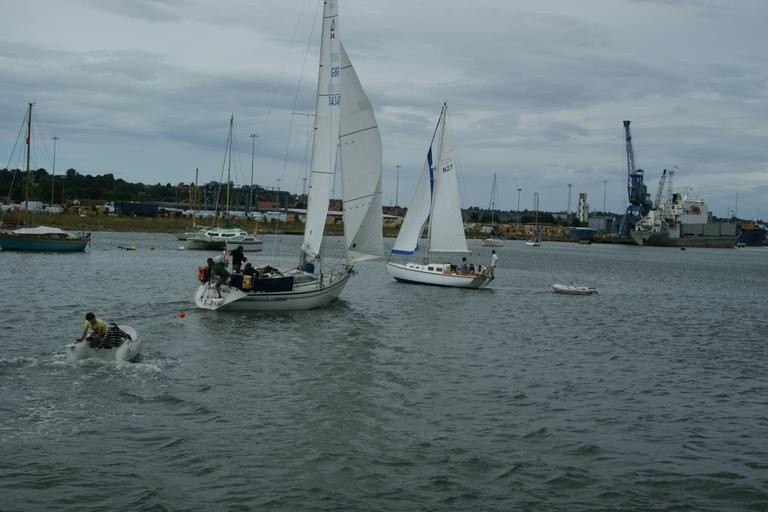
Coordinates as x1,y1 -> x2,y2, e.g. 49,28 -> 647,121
76,312 -> 132,352
206,258 -> 230,298
490,250 -> 498,267
229,245 -> 248,273
243,263 -> 260,276
219,249 -> 229,268
460,256 -> 470,273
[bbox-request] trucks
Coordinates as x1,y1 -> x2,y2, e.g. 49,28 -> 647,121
114,202 -> 170,218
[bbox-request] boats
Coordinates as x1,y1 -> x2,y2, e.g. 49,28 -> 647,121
630,199 -> 768,248
0,102 -> 91,252
526,239 -> 544,247
552,281 -> 596,297
66,325 -> 142,364
482,238 -> 505,249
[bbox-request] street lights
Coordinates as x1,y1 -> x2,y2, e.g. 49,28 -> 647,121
395,164 -> 401,206
517,189 -> 522,214
568,183 -> 572,214
603,179 -> 608,212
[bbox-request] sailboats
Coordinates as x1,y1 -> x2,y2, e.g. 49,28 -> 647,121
387,100 -> 495,290
